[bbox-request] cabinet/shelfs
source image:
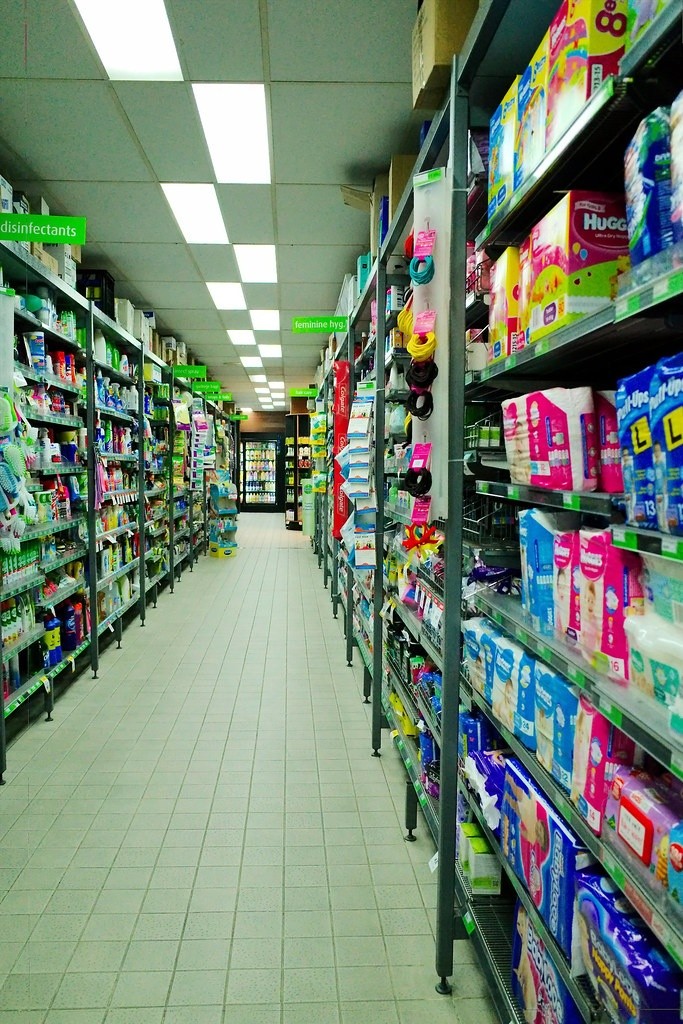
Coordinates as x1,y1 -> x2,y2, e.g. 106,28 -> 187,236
311,0 -> 683,1024
209,470 -> 237,559
0,240 -> 237,786
284,413 -> 310,531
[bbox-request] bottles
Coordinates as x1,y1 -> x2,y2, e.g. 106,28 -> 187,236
79,427 -> 88,451
152,424 -> 167,438
101,536 -> 133,578
239,461 -> 276,471
39,284 -> 86,349
1,591 -> 36,645
108,461 -> 138,491
284,488 -> 294,503
240,492 -> 275,505
95,326 -> 135,376
95,503 -> 140,534
98,575 -> 132,621
240,450 -> 276,462
239,482 -> 277,492
284,458 -> 293,467
94,417 -> 134,454
94,369 -> 139,413
41,431 -> 53,461
41,593 -> 91,673
240,442 -> 277,452
239,471 -> 277,481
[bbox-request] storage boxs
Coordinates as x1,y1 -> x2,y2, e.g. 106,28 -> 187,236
76,269 -> 116,322
529,189 -> 631,345
459,822 -> 487,877
118,299 -> 135,336
313,272 -> 353,388
134,309 -> 237,415
347,275 -> 358,317
487,75 -> 524,223
290,397 -> 308,413
544,0 -> 627,154
512,28 -> 550,192
468,838 -> 502,895
356,255 -> 369,299
378,196 -> 389,248
516,232 -> 531,352
0,175 -> 82,290
338,172 -> 389,265
387,153 -> 417,229
486,246 -> 520,367
411,0 -> 479,111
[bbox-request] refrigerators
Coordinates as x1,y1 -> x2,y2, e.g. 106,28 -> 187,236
239,430 -> 286,514
